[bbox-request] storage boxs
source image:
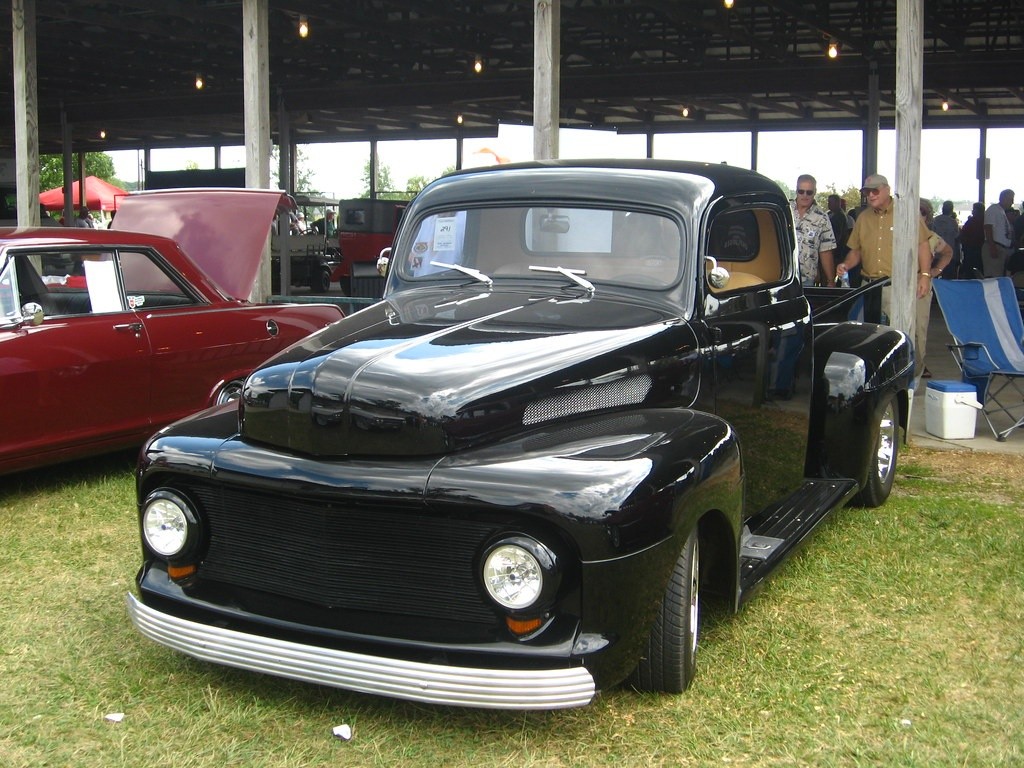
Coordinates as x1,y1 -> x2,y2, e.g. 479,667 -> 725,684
924,380 -> 983,440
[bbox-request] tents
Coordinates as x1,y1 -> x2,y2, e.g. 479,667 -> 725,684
38,175 -> 129,210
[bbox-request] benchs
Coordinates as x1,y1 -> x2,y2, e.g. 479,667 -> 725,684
491,259 -> 765,294
21,291 -> 193,315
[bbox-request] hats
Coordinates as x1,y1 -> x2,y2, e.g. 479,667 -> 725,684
326,209 -> 336,214
860,175 -> 887,191
80,207 -> 89,217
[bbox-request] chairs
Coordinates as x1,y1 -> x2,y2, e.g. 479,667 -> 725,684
930,277 -> 1024,441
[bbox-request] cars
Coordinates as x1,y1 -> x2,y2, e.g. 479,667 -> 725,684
0,189 -> 344,474
132,159 -> 918,715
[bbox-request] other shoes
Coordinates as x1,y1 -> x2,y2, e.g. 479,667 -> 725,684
922,366 -> 931,378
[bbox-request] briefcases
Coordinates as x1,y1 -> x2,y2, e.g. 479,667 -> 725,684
925,380 -> 983,440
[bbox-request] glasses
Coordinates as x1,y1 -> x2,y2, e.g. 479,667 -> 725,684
863,188 -> 885,196
797,189 -> 814,195
921,211 -> 926,216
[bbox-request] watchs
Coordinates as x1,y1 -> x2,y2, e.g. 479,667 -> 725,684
934,267 -> 943,272
921,272 -> 932,278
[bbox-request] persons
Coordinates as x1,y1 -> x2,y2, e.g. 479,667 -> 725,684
920,198 -> 954,278
837,174 -> 932,378
268,209 -> 336,238
74,207 -> 95,228
932,201 -> 962,279
42,209 -> 51,218
788,175 -> 837,288
59,217 -> 65,225
985,189 -> 1016,277
840,198 -> 856,236
826,194 -> 848,263
962,202 -> 985,279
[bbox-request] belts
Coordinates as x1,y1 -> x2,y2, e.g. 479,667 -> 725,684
985,241 -> 1010,250
863,277 -> 891,287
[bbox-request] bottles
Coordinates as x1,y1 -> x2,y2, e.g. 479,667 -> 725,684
839,263 -> 850,288
